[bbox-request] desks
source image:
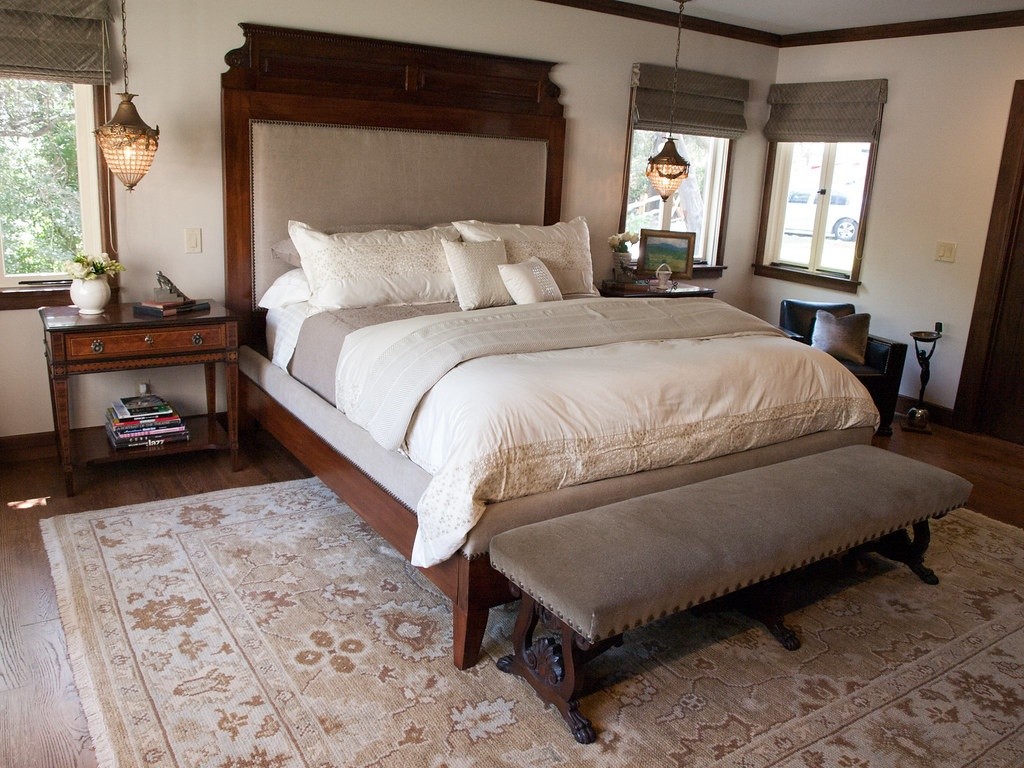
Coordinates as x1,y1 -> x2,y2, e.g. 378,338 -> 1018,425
39,298 -> 240,497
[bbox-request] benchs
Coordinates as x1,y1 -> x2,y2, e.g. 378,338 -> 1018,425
491,445 -> 973,744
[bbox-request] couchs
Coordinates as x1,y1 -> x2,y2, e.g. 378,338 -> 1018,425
775,300 -> 908,436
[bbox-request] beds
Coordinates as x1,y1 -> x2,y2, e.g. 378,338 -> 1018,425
221,22 -> 881,671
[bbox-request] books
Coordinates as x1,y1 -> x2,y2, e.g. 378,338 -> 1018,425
133,300 -> 211,318
105,395 -> 192,450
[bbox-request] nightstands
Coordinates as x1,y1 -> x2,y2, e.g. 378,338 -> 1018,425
603,279 -> 717,298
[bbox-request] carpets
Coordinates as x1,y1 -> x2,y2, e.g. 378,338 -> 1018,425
39,476 -> 1024,768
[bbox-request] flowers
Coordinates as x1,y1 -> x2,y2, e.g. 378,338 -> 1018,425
71,249 -> 127,281
607,231 -> 639,253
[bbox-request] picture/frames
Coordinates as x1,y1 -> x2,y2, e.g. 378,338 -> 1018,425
637,229 -> 696,279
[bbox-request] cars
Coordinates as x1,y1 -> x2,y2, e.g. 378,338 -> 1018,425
783,191 -> 860,241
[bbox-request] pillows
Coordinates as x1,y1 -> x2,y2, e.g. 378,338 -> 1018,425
270,216 -> 595,311
811,310 -> 871,364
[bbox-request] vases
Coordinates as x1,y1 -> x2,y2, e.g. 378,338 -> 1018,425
69,279 -> 111,315
612,252 -> 631,280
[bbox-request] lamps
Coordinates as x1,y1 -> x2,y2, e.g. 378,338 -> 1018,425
645,0 -> 690,204
92,0 -> 160,193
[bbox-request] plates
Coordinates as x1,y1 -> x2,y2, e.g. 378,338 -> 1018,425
910,331 -> 942,342
